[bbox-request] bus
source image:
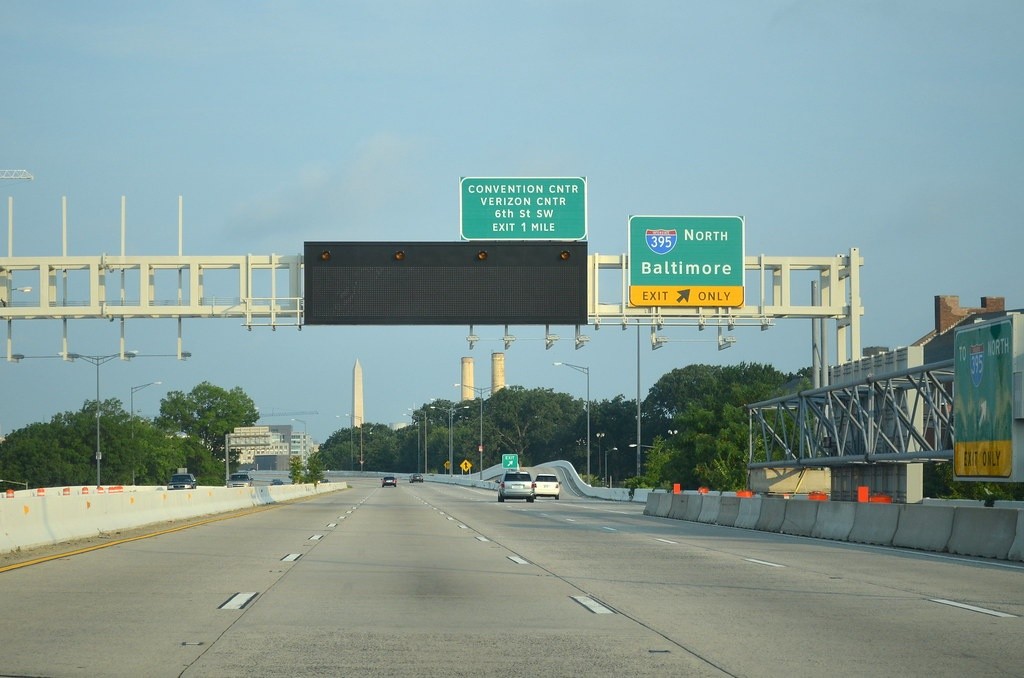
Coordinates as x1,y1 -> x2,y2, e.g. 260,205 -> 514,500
167,473 -> 197,490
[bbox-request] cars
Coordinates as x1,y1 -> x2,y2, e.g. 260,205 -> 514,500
381,476 -> 397,487
271,479 -> 284,485
410,474 -> 424,483
533,474 -> 562,500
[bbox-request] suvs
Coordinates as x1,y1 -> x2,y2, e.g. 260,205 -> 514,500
226,473 -> 254,487
494,469 -> 535,501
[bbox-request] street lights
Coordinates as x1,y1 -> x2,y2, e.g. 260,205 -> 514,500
130,381 -> 162,486
292,418 -> 308,481
604,448 -> 618,487
403,400 -> 470,478
552,362 -> 592,488
57,351 -> 139,487
596,433 -> 605,481
453,383 -> 507,483
337,414 -> 364,471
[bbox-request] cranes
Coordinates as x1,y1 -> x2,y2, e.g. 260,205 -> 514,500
258,408 -> 320,418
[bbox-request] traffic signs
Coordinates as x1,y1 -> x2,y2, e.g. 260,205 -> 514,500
948,314 -> 1014,481
626,215 -> 745,309
